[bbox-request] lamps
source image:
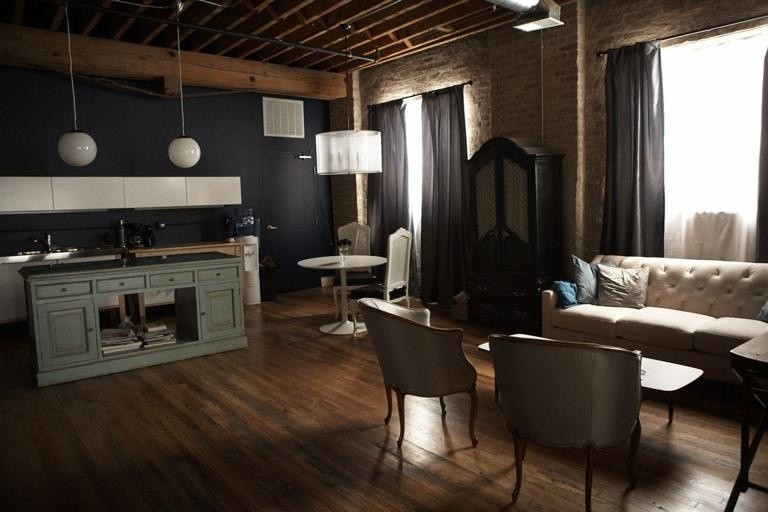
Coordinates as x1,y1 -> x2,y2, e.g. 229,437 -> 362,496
57,0 -> 98,168
168,0 -> 202,169
314,24 -> 384,176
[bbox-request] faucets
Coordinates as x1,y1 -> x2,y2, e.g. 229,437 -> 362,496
47,233 -> 53,252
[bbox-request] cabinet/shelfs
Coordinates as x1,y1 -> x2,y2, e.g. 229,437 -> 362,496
27,258 -> 247,389
463,135 -> 566,324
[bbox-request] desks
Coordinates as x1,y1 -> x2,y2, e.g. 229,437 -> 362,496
297,255 -> 387,336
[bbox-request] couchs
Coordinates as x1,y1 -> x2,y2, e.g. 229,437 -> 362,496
541,255 -> 767,392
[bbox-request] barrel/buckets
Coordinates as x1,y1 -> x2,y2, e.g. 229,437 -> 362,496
235,208 -> 256,236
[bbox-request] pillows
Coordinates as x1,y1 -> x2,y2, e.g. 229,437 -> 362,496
552,253 -> 649,308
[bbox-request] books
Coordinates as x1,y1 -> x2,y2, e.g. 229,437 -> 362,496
98,319 -> 177,357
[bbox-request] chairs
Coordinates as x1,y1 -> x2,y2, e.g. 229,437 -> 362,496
332,222 -> 413,335
